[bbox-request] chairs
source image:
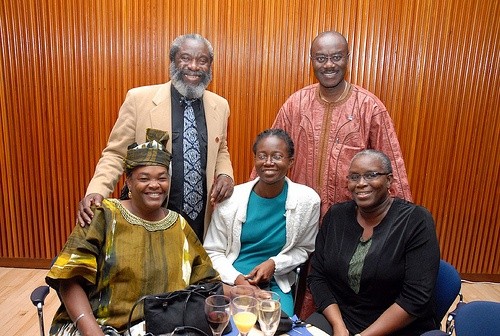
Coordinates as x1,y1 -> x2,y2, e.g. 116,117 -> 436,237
32,259 -> 500,336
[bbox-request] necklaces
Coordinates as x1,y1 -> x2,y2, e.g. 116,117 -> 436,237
320,81 -> 348,102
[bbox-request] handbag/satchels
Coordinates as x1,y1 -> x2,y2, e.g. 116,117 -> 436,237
128,281 -> 232,336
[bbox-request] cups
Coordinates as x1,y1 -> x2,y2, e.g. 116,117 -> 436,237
230,285 -> 255,302
256,291 -> 281,309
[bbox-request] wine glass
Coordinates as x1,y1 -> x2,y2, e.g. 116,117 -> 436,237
204,295 -> 281,336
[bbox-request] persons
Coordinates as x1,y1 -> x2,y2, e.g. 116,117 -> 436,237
76,34 -> 235,244
305,149 -> 441,336
249,30 -> 413,322
203,128 -> 321,318
45,128 -> 255,336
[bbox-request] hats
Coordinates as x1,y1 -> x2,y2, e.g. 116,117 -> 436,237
124,129 -> 172,170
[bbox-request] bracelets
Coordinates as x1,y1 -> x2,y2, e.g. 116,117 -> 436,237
73,312 -> 93,327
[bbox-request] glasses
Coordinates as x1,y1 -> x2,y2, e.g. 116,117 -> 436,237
345,172 -> 391,182
310,54 -> 349,64
254,155 -> 289,162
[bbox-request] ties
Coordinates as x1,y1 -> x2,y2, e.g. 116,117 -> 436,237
180,97 -> 203,220
